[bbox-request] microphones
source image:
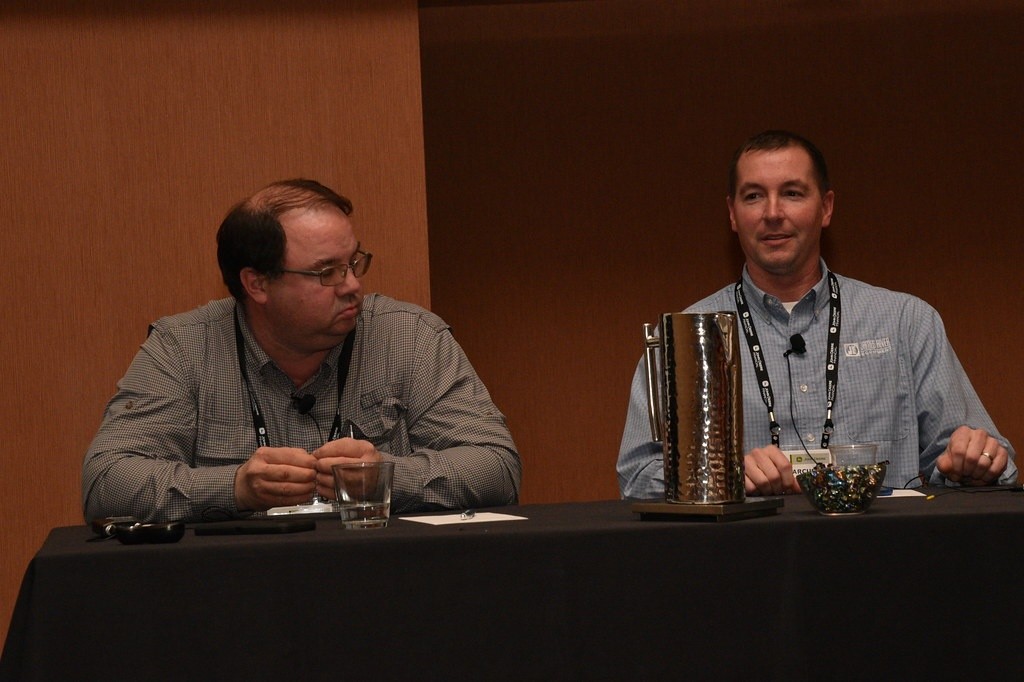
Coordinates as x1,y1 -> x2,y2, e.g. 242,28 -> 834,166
790,333 -> 807,355
298,394 -> 316,414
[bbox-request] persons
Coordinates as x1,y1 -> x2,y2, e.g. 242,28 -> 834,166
81,178 -> 523,526
615,129 -> 1018,501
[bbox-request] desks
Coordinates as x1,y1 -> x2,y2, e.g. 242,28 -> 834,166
0,486 -> 1024,682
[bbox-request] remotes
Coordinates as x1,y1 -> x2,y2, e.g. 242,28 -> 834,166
116,521 -> 185,544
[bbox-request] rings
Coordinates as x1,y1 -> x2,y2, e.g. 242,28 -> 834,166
981,452 -> 993,460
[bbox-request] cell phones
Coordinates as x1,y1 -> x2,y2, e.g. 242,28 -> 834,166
194,522 -> 316,535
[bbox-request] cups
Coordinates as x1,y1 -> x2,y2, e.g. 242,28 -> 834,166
829,444 -> 876,466
331,462 -> 395,530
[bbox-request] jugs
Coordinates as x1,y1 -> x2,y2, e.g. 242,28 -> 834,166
643,311 -> 745,504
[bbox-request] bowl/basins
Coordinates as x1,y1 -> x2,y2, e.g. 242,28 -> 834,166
797,464 -> 886,515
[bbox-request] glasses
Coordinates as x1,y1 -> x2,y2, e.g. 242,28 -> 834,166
274,249 -> 373,286
